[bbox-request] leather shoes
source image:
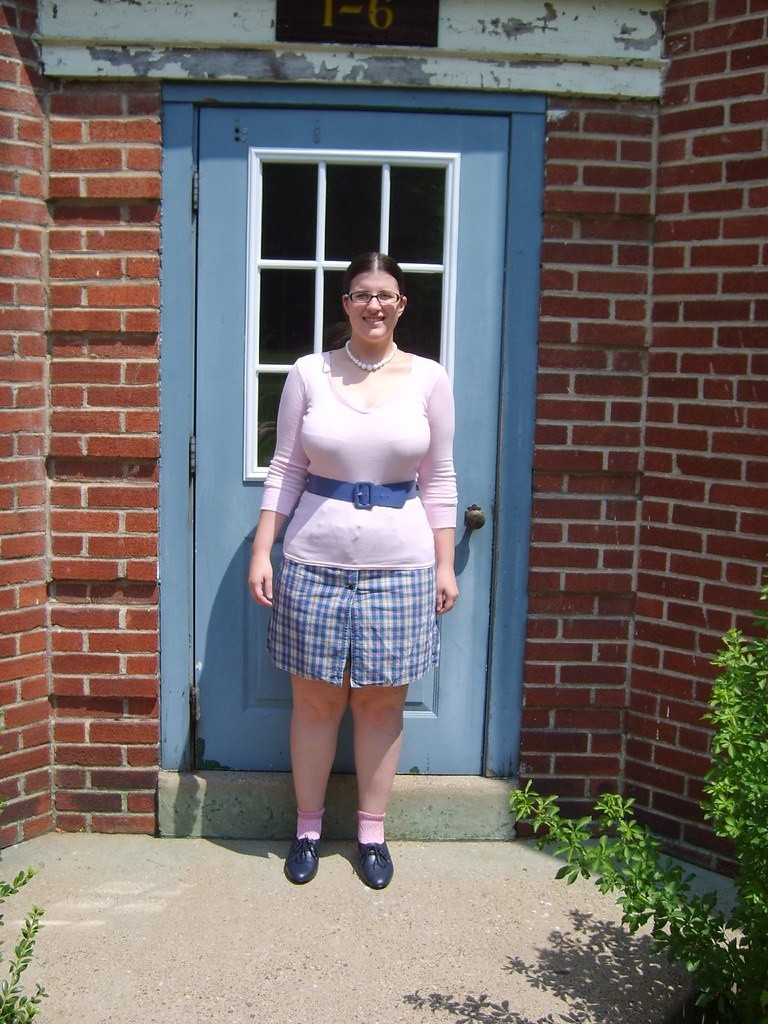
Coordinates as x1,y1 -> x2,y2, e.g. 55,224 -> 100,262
357,835 -> 394,890
284,830 -> 322,885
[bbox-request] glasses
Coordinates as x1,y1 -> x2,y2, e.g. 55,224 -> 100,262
348,290 -> 404,303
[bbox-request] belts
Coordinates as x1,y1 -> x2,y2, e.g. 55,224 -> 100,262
306,472 -> 417,509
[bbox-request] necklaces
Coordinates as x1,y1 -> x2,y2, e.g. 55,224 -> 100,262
346,339 -> 397,371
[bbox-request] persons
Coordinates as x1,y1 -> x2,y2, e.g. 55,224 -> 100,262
249,251 -> 460,890
